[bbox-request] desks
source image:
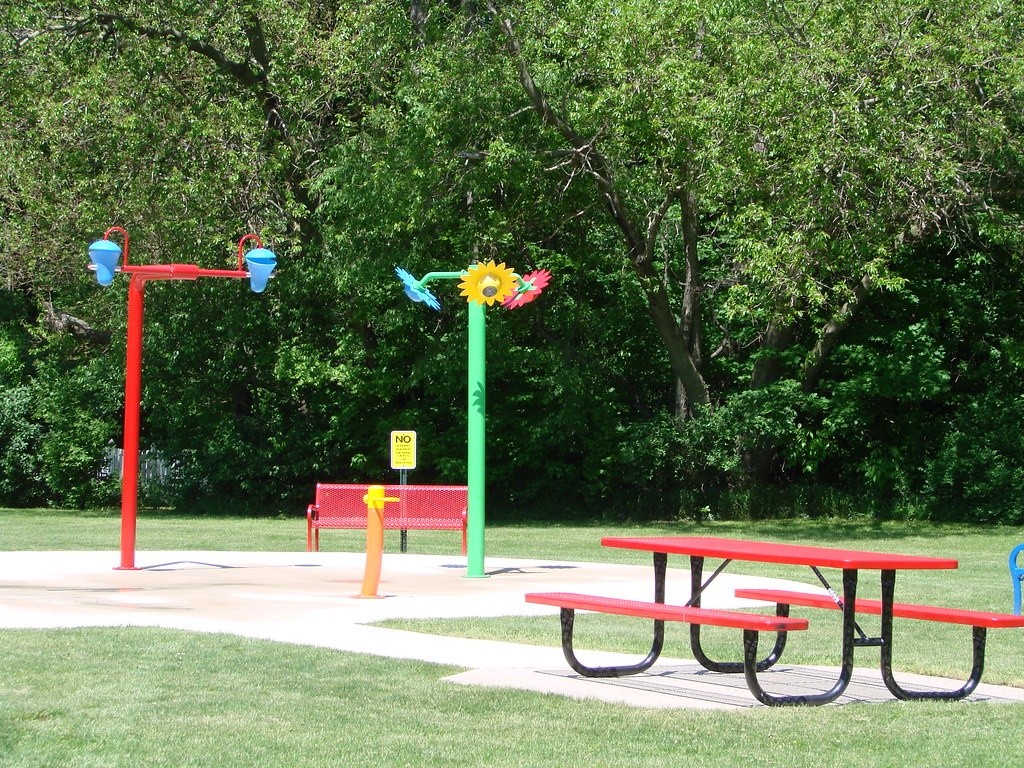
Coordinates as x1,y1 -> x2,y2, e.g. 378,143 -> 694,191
600,534 -> 959,702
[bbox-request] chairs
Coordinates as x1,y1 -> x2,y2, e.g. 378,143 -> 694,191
1009,544 -> 1024,616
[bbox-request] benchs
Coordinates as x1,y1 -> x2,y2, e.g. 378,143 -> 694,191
525,589 -> 1024,711
305,483 -> 469,557
